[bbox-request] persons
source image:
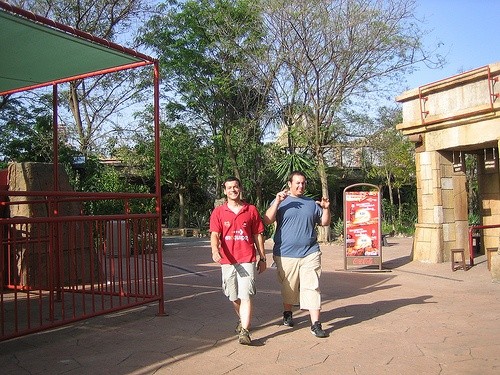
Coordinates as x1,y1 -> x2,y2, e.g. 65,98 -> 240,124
263,171 -> 331,338
209,176 -> 267,345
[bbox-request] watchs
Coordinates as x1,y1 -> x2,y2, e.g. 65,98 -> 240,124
260,258 -> 267,262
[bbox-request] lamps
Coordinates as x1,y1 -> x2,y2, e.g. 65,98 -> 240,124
409,135 -> 423,144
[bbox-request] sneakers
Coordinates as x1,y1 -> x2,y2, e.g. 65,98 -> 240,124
235,319 -> 242,334
283,311 -> 294,326
311,321 -> 326,336
238,327 -> 251,344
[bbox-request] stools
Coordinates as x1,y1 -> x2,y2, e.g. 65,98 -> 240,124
451,248 -> 467,272
486,247 -> 498,271
